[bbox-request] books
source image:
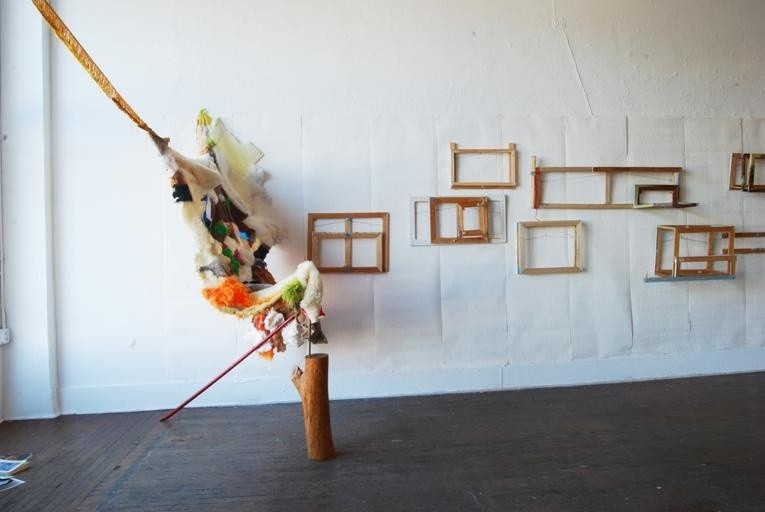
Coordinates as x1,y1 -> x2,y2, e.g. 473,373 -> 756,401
0,452 -> 33,476
